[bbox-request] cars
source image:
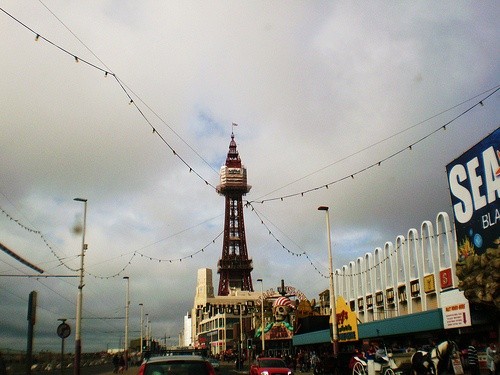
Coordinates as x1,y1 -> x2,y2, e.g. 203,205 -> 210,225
137,354 -> 215,375
250,356 -> 293,375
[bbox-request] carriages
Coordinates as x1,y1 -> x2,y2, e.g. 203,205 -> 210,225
353,340 -> 457,375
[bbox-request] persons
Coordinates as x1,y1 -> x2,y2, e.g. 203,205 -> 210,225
111,350 -> 147,375
486,341 -> 500,375
205,353 -> 261,371
466,340 -> 479,375
280,349 -> 321,373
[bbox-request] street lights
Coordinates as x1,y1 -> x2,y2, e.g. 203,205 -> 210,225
145,311 -> 153,354
74,196 -> 88,375
317,205 -> 339,360
122,275 -> 130,370
256,277 -> 265,356
139,302 -> 143,358
